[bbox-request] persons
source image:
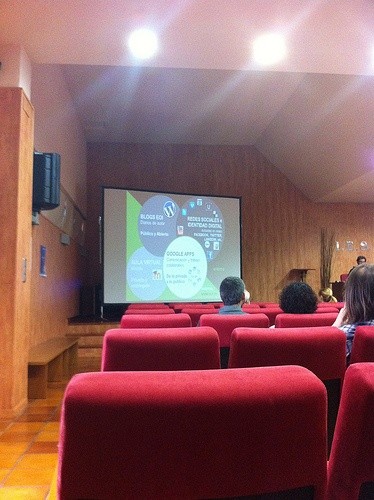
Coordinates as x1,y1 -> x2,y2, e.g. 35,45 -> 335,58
197,277 -> 249,327
318,287 -> 338,302
269,281 -> 318,328
331,264 -> 374,363
347,256 -> 366,278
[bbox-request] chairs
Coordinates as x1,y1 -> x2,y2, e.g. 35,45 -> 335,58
340,274 -> 349,281
58,302 -> 374,500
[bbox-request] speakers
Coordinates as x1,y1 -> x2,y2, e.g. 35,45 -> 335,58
32,152 -> 61,212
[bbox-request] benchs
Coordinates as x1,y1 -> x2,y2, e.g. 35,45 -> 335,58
28,336 -> 82,398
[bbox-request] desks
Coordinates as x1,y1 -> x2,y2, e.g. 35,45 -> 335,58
329,281 -> 346,301
